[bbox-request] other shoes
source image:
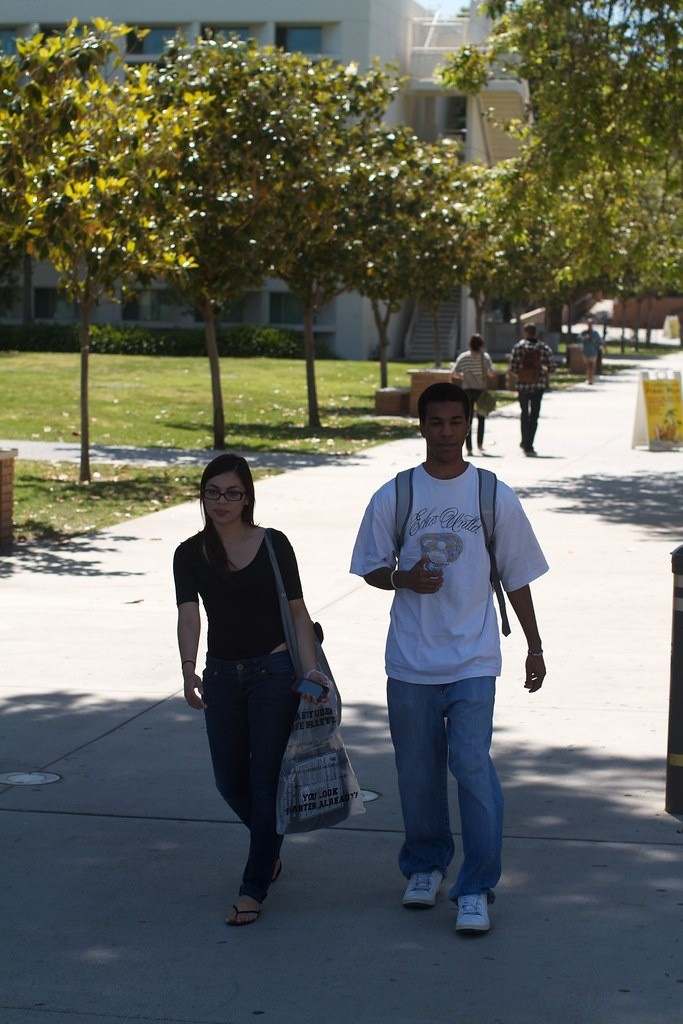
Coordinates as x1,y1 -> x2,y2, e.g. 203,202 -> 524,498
523,447 -> 536,456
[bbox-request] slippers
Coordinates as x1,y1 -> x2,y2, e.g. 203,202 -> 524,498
270,856 -> 282,882
224,904 -> 261,925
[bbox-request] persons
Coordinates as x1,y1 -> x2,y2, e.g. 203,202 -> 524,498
350,383 -> 549,933
172,454 -> 329,927
452,333 -> 496,456
509,323 -> 557,458
578,318 -> 608,386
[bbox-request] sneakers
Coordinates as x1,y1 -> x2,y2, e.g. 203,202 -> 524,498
452,889 -> 491,931
401,869 -> 443,905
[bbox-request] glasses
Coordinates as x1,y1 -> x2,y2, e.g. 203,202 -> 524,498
201,488 -> 247,501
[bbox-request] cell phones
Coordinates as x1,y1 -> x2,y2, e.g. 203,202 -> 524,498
292,676 -> 329,702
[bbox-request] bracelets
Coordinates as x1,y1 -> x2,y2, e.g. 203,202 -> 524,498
181,660 -> 196,670
527,650 -> 544,657
391,570 -> 404,592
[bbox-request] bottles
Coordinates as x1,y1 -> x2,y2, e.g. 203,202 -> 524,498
423,542 -> 448,579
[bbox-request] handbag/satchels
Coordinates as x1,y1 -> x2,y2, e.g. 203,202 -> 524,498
279,592 -> 342,727
276,694 -> 366,834
476,390 -> 496,417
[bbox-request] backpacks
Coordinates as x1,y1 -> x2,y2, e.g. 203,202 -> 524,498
515,347 -> 542,383
